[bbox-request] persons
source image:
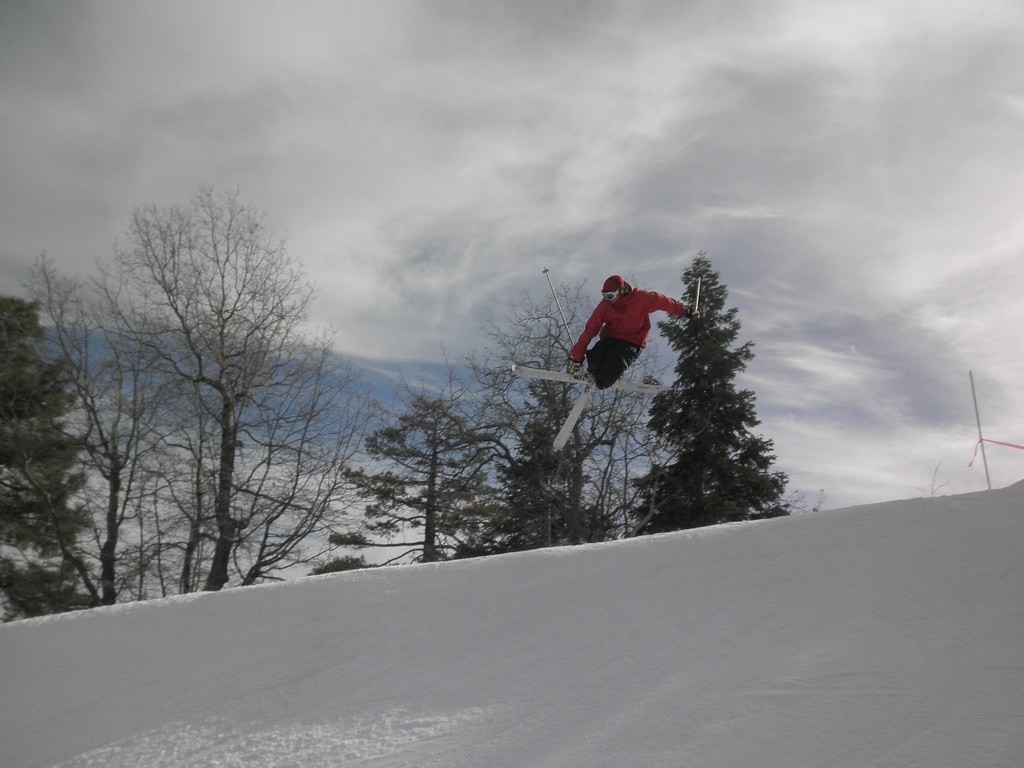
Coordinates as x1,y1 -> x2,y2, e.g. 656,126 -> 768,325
567,275 -> 700,390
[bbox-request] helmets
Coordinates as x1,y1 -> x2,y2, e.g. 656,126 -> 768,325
603,275 -> 624,292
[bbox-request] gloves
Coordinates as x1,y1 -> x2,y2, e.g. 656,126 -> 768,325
686,308 -> 701,320
572,362 -> 583,380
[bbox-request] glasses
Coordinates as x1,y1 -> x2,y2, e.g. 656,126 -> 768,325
602,292 -> 617,300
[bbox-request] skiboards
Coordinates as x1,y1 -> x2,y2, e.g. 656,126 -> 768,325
511,364 -> 673,450
